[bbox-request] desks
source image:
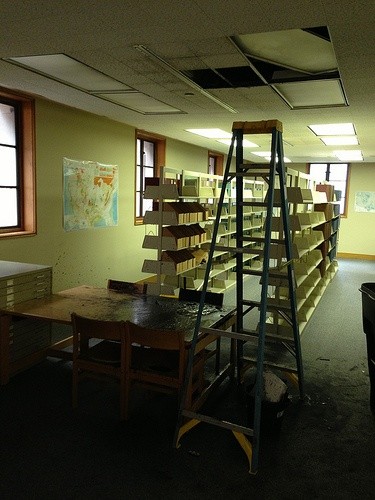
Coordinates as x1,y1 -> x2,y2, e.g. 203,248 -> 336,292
0,285 -> 238,387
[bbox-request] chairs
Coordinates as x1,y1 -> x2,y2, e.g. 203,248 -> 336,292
70,279 -> 223,418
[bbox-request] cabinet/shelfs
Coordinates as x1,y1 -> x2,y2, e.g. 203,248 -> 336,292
0,261 -> 53,386
139,174 -> 341,340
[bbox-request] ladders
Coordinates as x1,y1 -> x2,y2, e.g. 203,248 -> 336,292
166,118 -> 309,475
